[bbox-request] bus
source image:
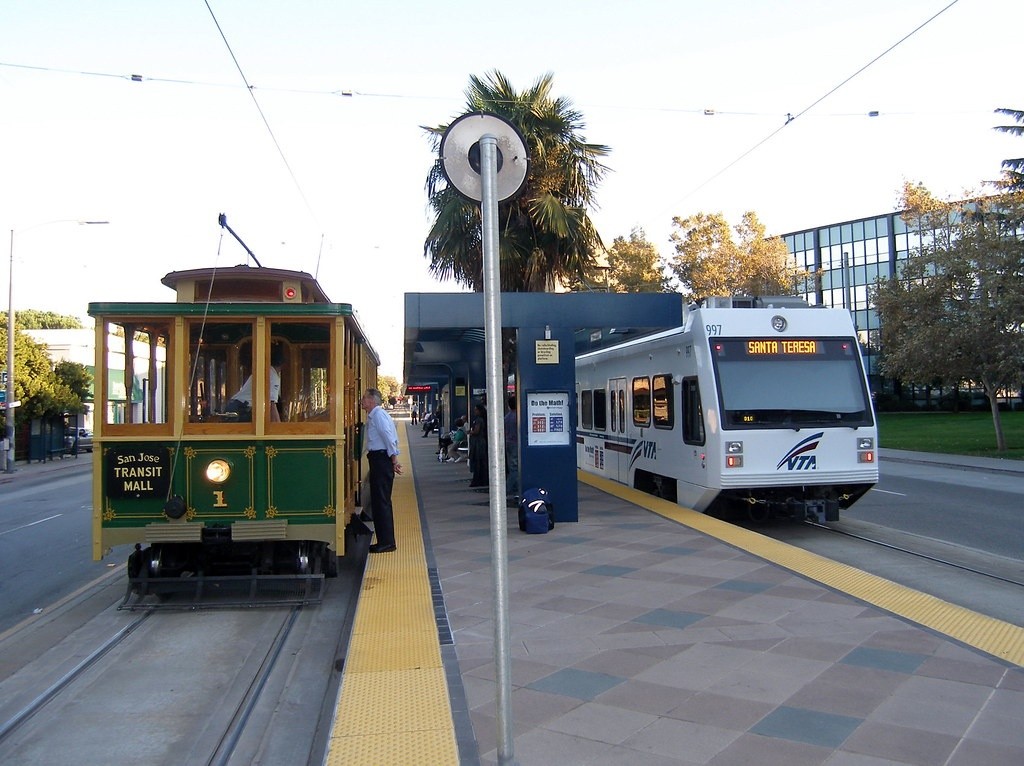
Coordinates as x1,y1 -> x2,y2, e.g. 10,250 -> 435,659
576,297 -> 881,525
85,214 -> 381,604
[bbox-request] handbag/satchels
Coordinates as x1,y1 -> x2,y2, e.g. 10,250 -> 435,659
458,440 -> 468,447
439,447 -> 447,460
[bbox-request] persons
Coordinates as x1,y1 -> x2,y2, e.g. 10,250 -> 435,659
223,353 -> 285,422
361,388 -> 403,553
466,393 -> 489,487
420,405 -> 444,438
437,416 -> 468,463
505,396 -> 518,496
411,402 -> 417,425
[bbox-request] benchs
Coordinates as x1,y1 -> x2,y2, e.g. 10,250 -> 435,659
455,447 -> 468,464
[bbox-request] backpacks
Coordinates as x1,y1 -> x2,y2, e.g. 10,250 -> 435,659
518,488 -> 554,534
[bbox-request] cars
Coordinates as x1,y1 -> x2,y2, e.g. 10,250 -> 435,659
67,427 -> 95,452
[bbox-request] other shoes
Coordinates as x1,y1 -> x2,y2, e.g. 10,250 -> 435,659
369,544 -> 378,550
455,456 -> 462,463
422,435 -> 428,438
446,457 -> 455,462
369,544 -> 396,552
436,450 -> 440,454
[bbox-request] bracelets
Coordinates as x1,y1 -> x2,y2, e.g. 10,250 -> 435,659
469,430 -> 470,433
393,462 -> 398,465
